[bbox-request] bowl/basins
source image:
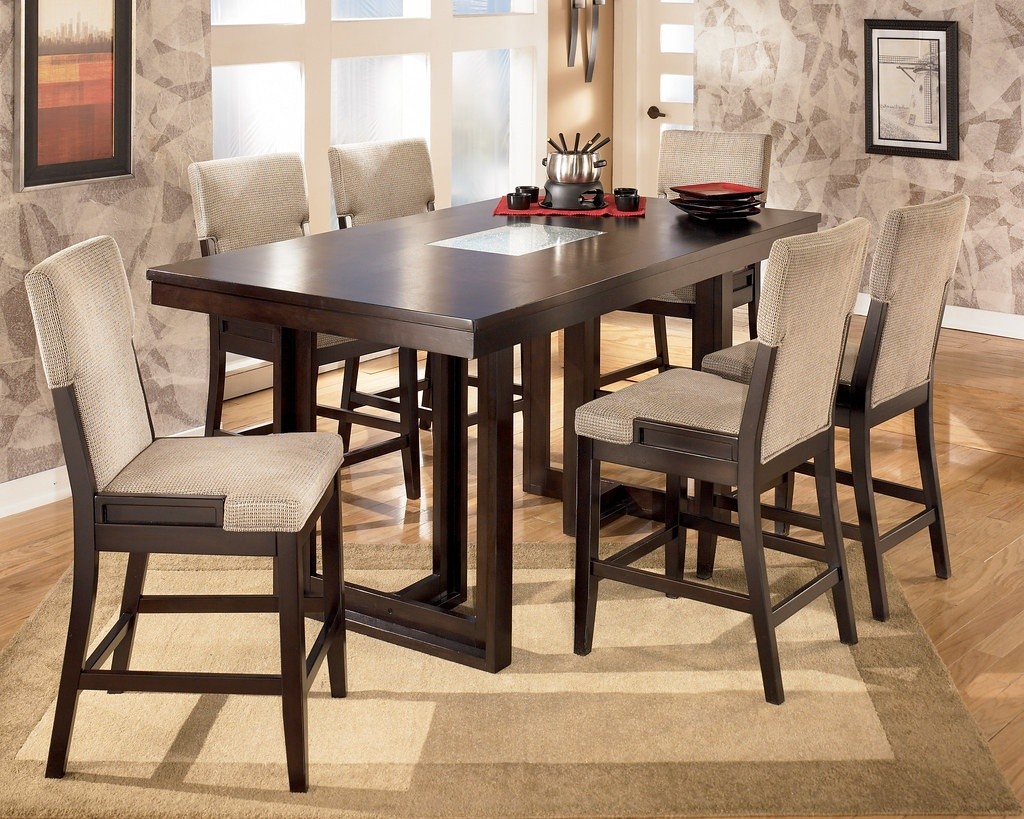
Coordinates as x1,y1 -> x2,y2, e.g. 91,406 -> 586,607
614,194 -> 640,212
613,188 -> 638,197
515,186 -> 539,203
506,192 -> 532,210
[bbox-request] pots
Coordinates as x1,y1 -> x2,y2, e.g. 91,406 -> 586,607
541,150 -> 607,184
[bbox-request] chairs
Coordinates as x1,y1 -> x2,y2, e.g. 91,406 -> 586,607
24,235 -> 344,793
574,216 -> 871,705
585,129 -> 772,402
328,139 -> 524,452
695,193 -> 971,622
188,151 -> 421,500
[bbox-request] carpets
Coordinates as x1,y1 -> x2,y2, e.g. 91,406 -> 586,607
0,540 -> 1024,819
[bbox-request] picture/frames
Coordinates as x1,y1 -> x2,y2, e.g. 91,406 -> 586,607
15,0 -> 137,192
864,19 -> 960,161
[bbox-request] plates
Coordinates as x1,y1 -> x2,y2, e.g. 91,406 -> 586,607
669,182 -> 765,222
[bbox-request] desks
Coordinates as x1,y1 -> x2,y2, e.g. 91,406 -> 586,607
146,190 -> 822,673
